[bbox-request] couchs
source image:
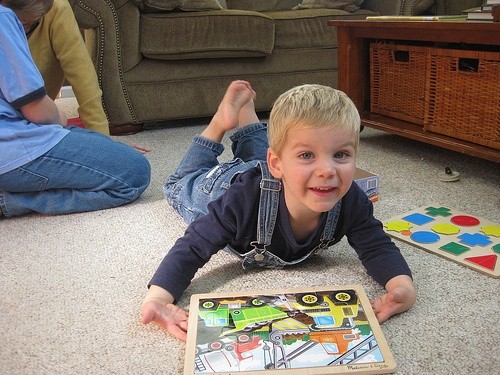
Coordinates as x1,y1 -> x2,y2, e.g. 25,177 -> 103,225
71,0 -> 488,136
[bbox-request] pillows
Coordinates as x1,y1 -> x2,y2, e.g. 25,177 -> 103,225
144,0 -> 366,13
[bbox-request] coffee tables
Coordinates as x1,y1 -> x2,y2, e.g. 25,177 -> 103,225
327,17 -> 500,164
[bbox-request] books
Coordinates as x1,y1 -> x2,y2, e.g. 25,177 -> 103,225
460,0 -> 500,21
366,15 -> 468,21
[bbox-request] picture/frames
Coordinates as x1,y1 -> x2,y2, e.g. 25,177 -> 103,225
184,284 -> 396,375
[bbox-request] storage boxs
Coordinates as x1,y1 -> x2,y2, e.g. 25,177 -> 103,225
354,166 -> 379,203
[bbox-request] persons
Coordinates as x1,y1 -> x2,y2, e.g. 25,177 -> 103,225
0,0 -> 152,220
142,80 -> 415,341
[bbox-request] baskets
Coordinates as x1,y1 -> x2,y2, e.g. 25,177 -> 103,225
368,41 -> 429,126
423,46 -> 500,148
